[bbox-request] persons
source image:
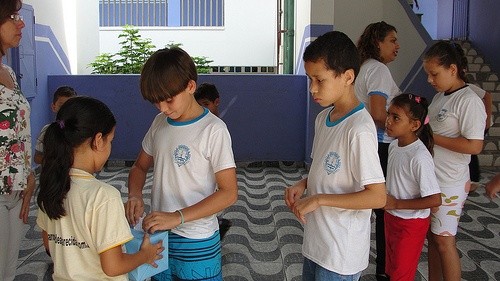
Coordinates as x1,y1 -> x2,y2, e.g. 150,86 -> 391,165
285,31 -> 387,281
422,40 -> 487,281
34,97 -> 165,281
485,171 -> 500,199
383,91 -> 442,281
194,83 -> 232,240
354,21 -> 404,279
34,86 -> 77,163
0,0 -> 35,281
125,47 -> 238,281
467,82 -> 492,191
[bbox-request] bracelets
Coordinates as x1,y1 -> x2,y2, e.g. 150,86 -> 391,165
176,210 -> 184,225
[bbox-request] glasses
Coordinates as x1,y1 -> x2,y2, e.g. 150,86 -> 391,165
6,13 -> 25,23
